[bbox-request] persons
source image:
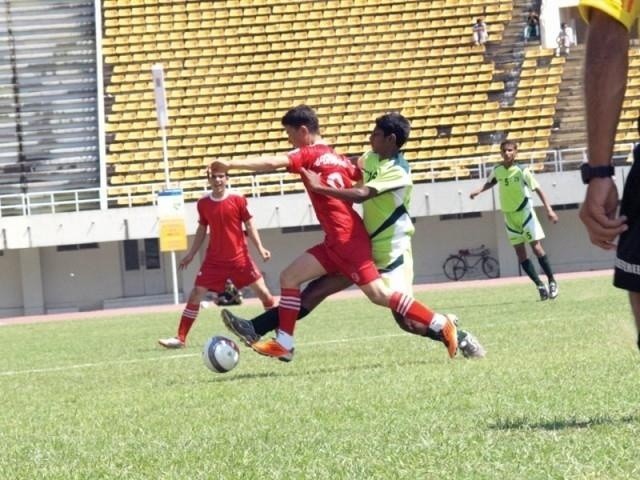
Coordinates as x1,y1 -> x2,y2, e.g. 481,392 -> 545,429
470,140 -> 560,300
579,0 -> 640,353
158,161 -> 278,348
523,8 -> 539,45
216,279 -> 243,305
556,22 -> 574,46
220,113 -> 487,361
472,19 -> 489,45
202,104 -> 458,362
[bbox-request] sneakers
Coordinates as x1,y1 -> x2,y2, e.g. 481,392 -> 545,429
457,330 -> 484,359
549,281 -> 559,298
251,339 -> 294,361
437,313 -> 459,359
159,336 -> 184,348
221,309 -> 262,347
537,284 -> 548,301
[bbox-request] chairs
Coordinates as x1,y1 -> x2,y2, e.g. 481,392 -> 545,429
610,46 -> 640,152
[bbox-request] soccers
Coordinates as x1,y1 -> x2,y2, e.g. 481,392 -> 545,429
203,335 -> 239,372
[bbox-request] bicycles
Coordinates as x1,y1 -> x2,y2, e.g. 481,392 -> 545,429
439,245 -> 506,285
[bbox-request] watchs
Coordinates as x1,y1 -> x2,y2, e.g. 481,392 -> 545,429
581,163 -> 614,184
